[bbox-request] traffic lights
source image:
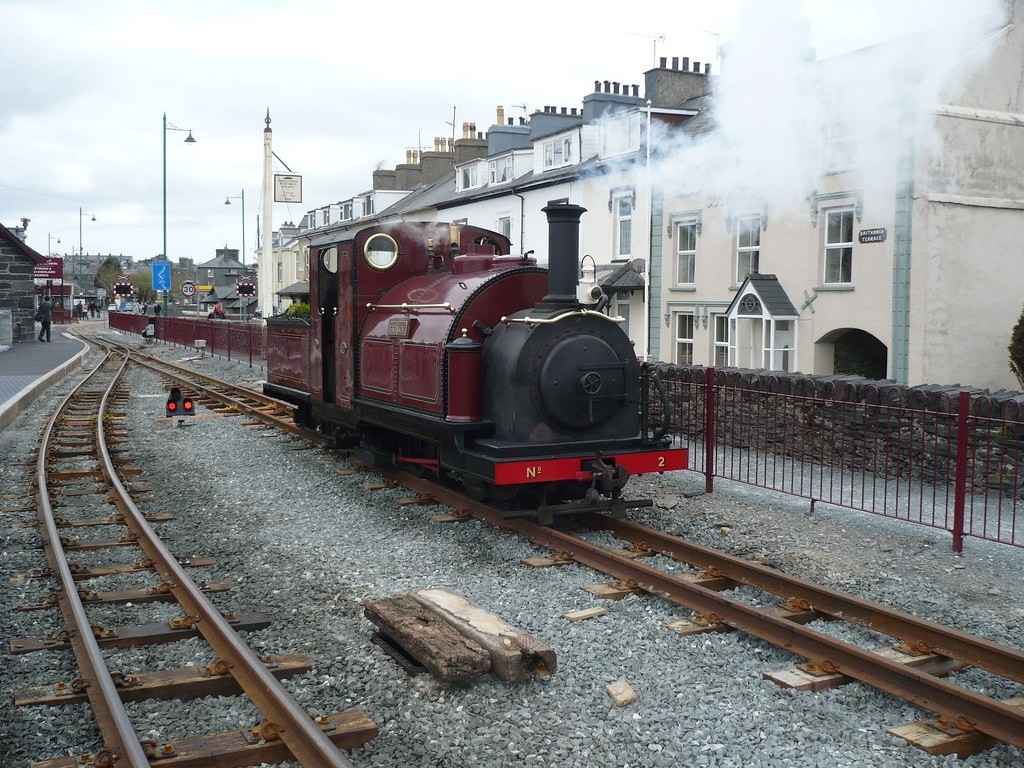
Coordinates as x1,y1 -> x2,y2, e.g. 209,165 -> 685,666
166,389 -> 194,417
163,113 -> 197,316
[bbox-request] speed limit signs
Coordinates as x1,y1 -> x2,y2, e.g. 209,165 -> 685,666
181,283 -> 196,296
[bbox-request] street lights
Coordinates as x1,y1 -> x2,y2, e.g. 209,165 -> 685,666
80,208 -> 96,278
225,188 -> 246,322
49,232 -> 61,260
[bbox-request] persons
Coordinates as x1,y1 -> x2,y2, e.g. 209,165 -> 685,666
207,306 -> 226,320
82,303 -> 89,320
87,301 -> 101,318
38,296 -> 51,342
153,301 -> 161,317
78,301 -> 82,319
134,303 -> 148,314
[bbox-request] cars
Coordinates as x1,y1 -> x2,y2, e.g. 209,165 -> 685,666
108,304 -> 116,311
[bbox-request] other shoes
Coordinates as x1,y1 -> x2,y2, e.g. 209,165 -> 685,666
47,339 -> 51,342
38,337 -> 46,342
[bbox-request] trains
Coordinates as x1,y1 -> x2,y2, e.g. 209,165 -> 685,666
263,195 -> 689,516
